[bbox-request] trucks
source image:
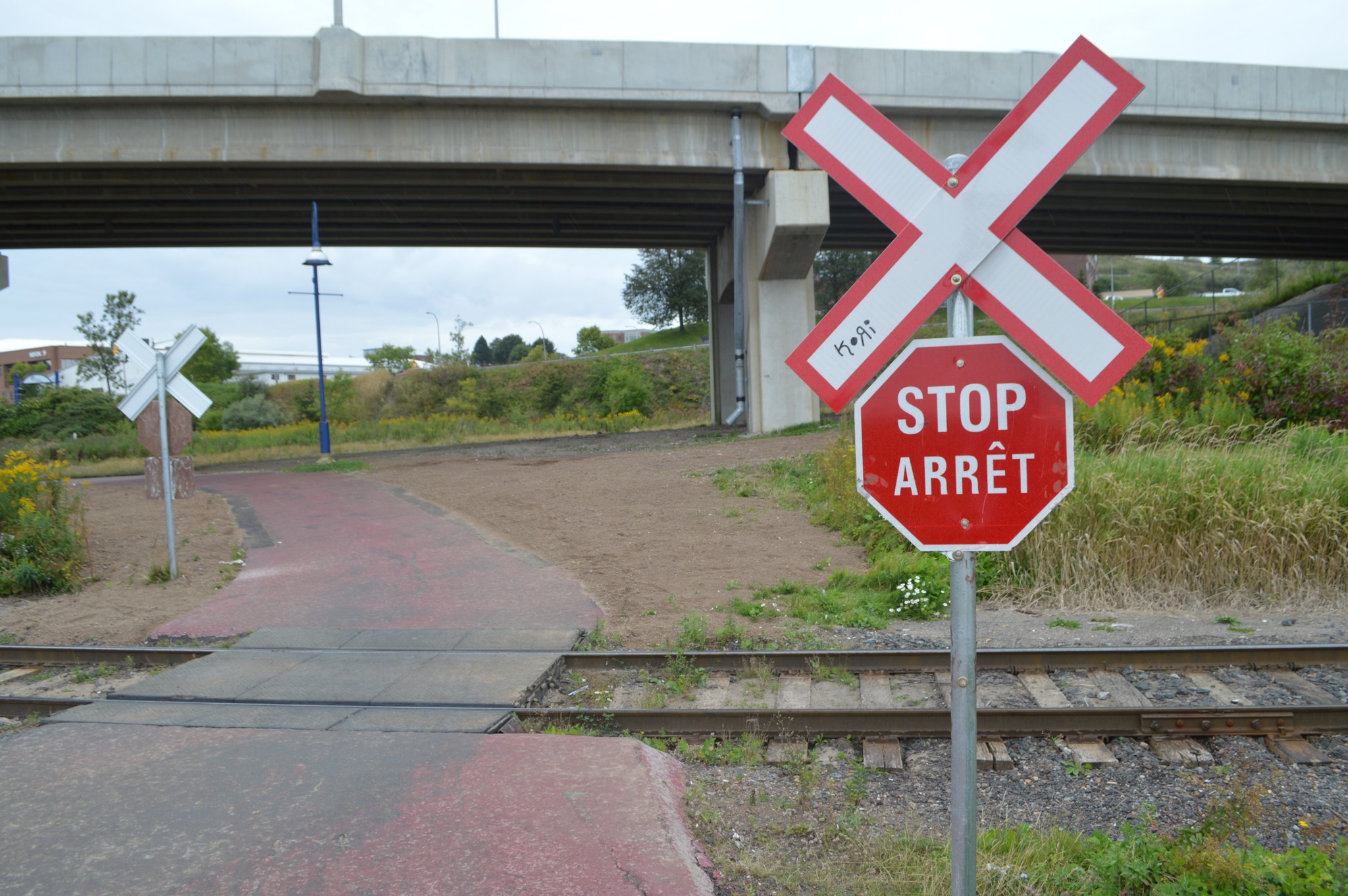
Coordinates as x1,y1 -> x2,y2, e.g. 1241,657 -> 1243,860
1203,288 -> 1245,297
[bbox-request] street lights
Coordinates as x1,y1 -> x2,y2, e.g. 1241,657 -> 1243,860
302,200 -> 336,463
426,311 -> 442,368
530,320 -> 547,363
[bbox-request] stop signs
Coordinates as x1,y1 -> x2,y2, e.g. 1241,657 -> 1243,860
853,336 -> 1075,551
